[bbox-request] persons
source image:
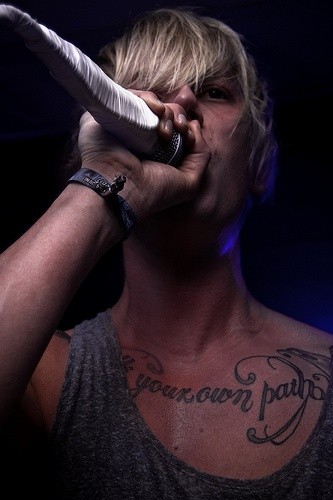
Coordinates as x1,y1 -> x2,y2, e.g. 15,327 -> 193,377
0,7 -> 333,500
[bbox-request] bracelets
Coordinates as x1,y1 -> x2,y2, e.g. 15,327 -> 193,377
67,167 -> 139,241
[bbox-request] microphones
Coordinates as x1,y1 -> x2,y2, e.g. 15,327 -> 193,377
54,31 -> 186,166
